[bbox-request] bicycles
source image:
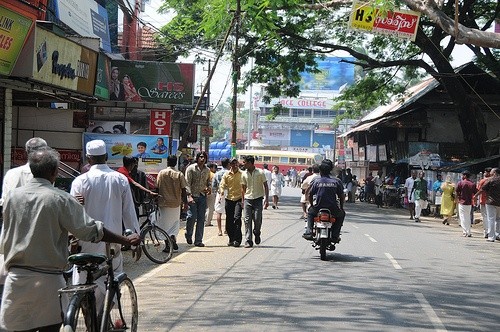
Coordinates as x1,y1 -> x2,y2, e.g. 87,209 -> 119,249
57,228 -> 143,332
120,194 -> 173,264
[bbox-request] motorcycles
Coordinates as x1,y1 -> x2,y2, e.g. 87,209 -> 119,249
301,190 -> 347,261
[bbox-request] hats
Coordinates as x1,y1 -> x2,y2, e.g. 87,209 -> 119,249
86,139 -> 106,155
230,158 -> 239,163
463,170 -> 471,178
123,155 -> 136,165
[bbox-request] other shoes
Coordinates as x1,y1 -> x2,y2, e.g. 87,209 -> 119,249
187,239 -> 192,244
228,241 -> 234,246
224,231 -> 227,234
272,205 -> 276,209
205,224 -> 213,226
164,244 -> 170,253
410,217 -> 413,220
234,241 -> 239,247
300,215 -> 304,218
194,242 -> 204,247
245,244 -> 253,248
415,219 -> 418,222
255,237 -> 260,244
443,218 -> 447,224
115,320 -> 124,331
302,233 -> 313,240
218,232 -> 222,236
337,238 -> 341,241
173,241 -> 179,253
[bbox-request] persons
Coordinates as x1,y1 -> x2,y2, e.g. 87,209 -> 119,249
280,166 -> 309,188
150,138 -> 167,154
69,138 -> 142,315
432,167 -> 500,242
157,155 -> 186,253
117,156 -> 158,221
91,125 -> 126,134
105,60 -> 142,102
263,164 -> 286,210
205,156 -> 269,248
0,147 -> 141,332
299,159 -> 346,243
185,151 -> 212,247
337,168 -> 358,203
404,170 -> 429,222
134,142 -> 150,158
0,137 -> 85,284
365,171 -> 395,208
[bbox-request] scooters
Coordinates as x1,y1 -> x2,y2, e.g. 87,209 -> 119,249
354,179 -> 431,217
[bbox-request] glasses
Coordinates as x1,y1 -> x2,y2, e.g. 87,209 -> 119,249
199,156 -> 206,159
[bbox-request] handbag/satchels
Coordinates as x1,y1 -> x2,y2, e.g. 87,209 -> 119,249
404,197 -> 408,205
451,187 -> 455,201
